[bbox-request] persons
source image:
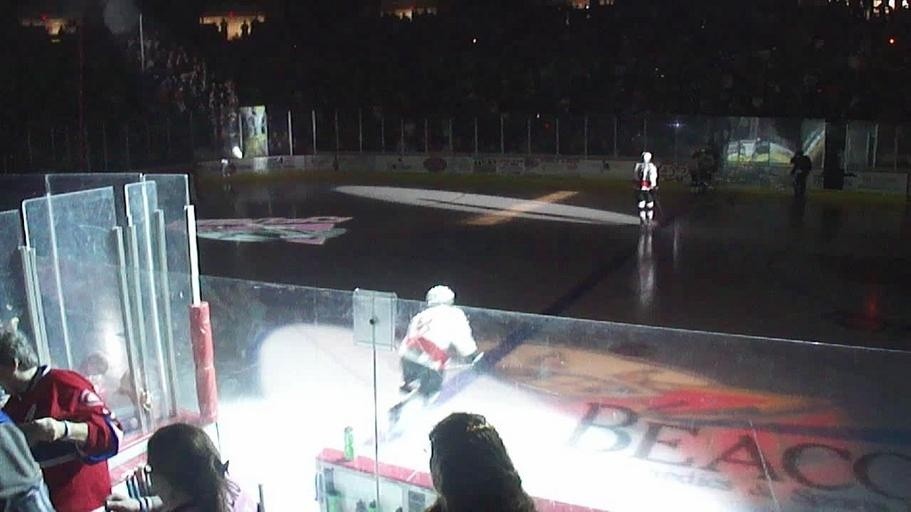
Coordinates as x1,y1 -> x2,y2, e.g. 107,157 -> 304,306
0,329 -> 124,512
0,410 -> 57,512
105,423 -> 241,512
422,413 -> 539,511
378,285 -> 488,446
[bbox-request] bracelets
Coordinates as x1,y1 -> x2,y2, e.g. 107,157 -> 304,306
60,420 -> 71,442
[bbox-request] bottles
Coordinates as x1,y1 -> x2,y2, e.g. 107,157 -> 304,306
328,489 -> 403,511
344,424 -> 355,460
